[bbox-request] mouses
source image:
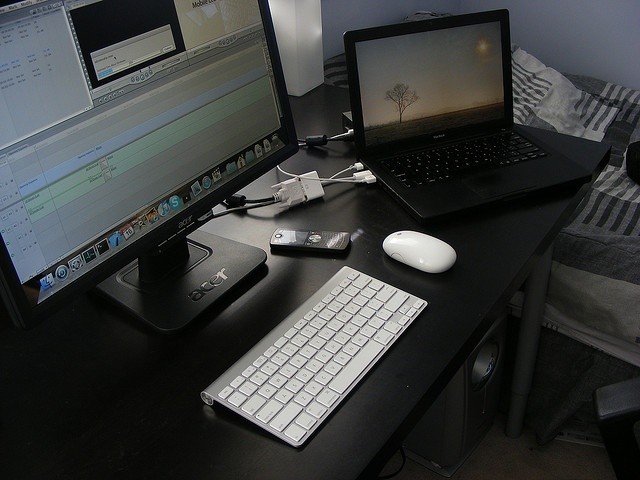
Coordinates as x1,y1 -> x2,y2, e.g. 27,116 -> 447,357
382,230 -> 457,274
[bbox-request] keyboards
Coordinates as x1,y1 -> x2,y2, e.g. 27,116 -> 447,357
200,265 -> 429,449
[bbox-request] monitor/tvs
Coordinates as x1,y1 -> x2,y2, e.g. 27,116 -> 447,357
1,0 -> 300,348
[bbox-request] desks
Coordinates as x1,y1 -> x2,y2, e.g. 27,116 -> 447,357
0,83 -> 611,480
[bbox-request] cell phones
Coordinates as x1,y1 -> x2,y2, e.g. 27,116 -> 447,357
269,226 -> 353,253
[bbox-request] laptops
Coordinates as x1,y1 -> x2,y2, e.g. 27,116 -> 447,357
342,9 -> 593,224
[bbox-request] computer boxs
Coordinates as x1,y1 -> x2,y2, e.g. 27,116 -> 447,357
389,306 -> 514,476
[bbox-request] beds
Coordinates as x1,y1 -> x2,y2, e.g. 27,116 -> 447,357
323,12 -> 640,367
